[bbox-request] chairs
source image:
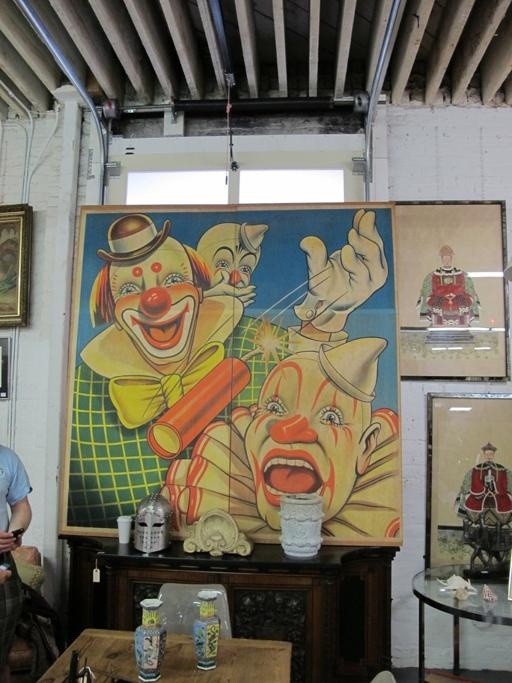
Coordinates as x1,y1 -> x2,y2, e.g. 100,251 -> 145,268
1,546 -> 59,683
156,582 -> 231,641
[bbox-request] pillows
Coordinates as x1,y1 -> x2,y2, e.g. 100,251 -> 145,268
13,559 -> 46,597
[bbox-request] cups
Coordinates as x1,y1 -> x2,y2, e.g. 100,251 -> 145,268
116,514 -> 133,544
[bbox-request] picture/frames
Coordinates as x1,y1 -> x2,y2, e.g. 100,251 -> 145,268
388,199 -> 512,384
424,391 -> 512,582
0,203 -> 34,328
56,200 -> 405,550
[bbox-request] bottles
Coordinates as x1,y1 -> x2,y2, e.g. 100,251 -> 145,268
135,587 -> 224,681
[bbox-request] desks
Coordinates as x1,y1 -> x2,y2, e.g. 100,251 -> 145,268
411,564 -> 512,682
36,629 -> 293,683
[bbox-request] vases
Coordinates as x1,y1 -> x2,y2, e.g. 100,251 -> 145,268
193,590 -> 221,671
134,599 -> 167,682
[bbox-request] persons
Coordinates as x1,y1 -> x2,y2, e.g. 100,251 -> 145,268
413,243 -> 482,328
369,668 -> 396,683
0,445 -> 43,595
453,440 -> 512,525
144,637 -> 153,663
0,567 -> 12,588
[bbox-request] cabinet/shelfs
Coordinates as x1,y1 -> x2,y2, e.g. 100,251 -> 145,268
57,535 -> 401,683
